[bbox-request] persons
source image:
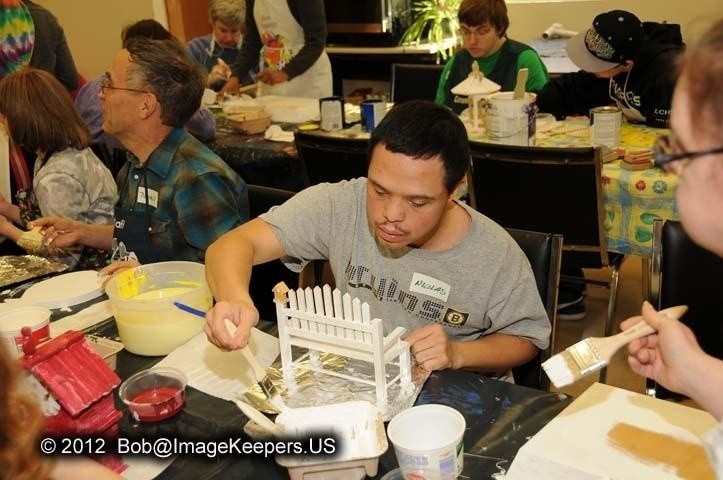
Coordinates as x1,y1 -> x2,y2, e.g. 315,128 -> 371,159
620,15 -> 723,480
0,0 -> 693,321
201,99 -> 556,384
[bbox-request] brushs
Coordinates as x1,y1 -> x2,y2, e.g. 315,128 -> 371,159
224,319 -> 286,410
541,305 -> 689,389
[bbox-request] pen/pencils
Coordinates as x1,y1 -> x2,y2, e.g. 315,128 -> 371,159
174,301 -> 207,317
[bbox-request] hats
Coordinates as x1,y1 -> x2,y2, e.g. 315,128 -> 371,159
566,10 -> 644,73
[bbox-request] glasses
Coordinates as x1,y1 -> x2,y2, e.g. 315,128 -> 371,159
102,77 -> 150,95
650,135 -> 722,176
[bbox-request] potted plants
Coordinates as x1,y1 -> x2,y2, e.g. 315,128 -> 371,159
395,0 -> 465,64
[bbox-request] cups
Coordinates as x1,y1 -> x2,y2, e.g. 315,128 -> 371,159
386,403 -> 466,480
119,368 -> 187,422
534,113 -> 552,132
0,307 -> 52,361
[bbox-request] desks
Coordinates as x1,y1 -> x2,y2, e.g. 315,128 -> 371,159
324,37 -> 463,108
525,37 -> 583,84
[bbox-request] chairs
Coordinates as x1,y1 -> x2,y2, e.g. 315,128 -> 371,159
0,64 -> 722,479
